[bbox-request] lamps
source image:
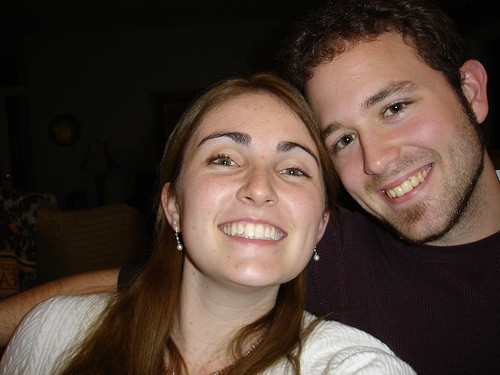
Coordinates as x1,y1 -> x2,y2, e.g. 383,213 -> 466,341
80,139 -> 121,206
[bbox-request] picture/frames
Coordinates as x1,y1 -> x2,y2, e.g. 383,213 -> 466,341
150,88 -> 205,161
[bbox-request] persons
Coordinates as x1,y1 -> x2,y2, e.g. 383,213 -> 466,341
0,0 -> 500,375
1,71 -> 417,375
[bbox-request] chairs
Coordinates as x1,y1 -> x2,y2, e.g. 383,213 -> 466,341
35,204 -> 152,284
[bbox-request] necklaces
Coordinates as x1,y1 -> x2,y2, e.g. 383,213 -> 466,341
213,335 -> 262,375
